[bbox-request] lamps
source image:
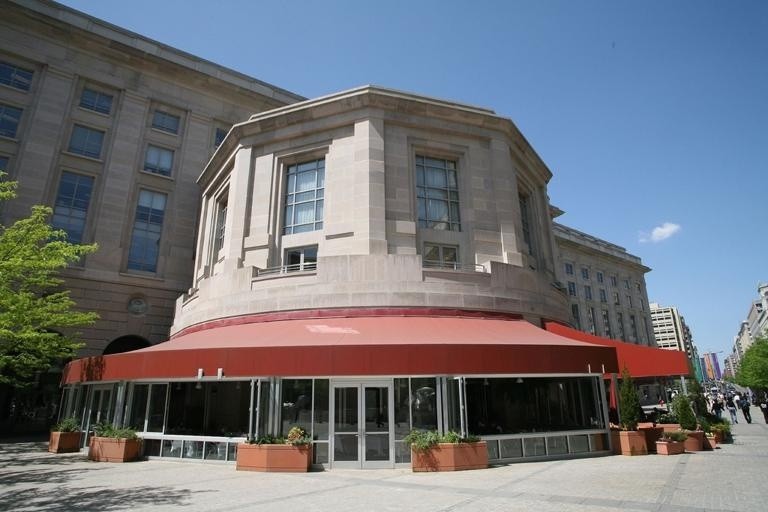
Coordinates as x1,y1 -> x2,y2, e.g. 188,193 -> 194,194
196,383 -> 203,390
516,377 -> 524,384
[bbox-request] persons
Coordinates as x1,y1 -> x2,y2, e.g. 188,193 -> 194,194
704,378 -> 768,424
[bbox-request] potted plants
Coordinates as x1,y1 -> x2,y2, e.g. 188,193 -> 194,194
404,428 -> 489,472
87,422 -> 146,463
611,367 -> 731,455
48,418 -> 82,454
235,427 -> 313,472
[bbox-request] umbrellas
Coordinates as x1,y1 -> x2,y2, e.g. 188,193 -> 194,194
609,376 -> 622,409
